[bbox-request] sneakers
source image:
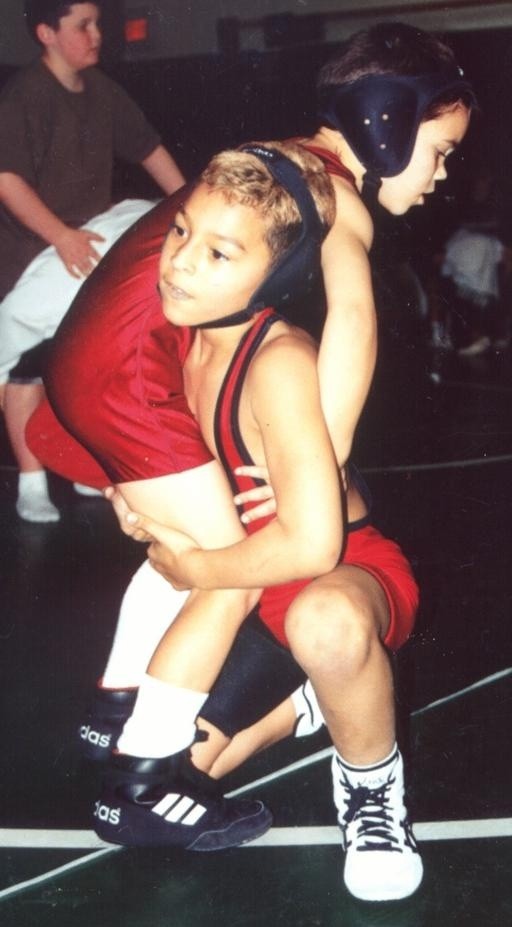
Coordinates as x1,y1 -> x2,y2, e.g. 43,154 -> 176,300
322,750 -> 426,904
86,736 -> 276,856
77,674 -> 214,762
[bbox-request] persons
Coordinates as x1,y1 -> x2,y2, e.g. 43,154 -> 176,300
0,2 -> 193,522
0,196 -> 159,491
46,20 -> 477,854
104,140 -> 426,902
